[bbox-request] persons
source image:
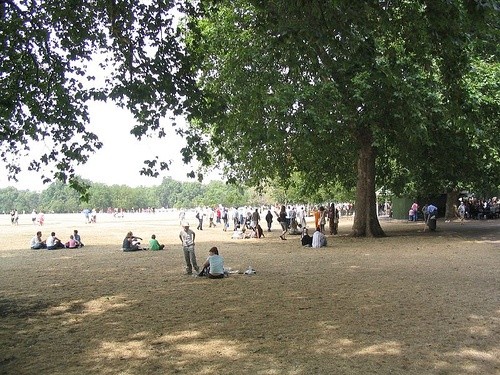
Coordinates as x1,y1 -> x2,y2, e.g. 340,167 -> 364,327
11,207 -> 96,250
203,247 -> 228,279
452,197 -> 500,225
179,221 -> 199,275
408,199 -> 438,232
148,235 -> 164,250
123,231 -> 143,251
179,200 -> 339,248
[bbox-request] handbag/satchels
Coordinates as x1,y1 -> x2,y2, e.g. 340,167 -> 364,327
277,217 -> 281,223
318,218 -> 322,225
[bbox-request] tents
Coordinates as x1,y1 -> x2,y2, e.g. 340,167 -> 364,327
391,177 -> 467,219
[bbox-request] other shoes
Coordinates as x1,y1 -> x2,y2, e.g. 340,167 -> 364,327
279,235 -> 287,241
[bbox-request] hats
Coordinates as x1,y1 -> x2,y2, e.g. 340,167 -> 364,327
183,221 -> 189,227
